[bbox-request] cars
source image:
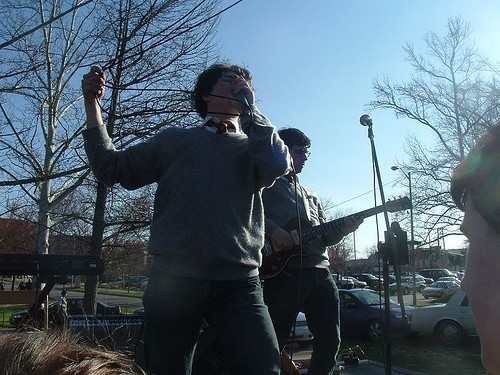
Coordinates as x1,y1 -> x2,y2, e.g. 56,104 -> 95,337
287,312 -> 314,350
331,269 -> 465,299
410,287 -> 478,349
9,297 -> 121,331
119,276 -> 150,291
339,287 -> 419,343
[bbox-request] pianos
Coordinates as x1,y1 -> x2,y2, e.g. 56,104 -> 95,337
0,253 -> 105,277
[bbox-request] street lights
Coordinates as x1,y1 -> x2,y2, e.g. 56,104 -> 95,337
391,166 -> 417,306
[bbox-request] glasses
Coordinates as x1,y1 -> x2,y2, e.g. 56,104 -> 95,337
290,147 -> 311,158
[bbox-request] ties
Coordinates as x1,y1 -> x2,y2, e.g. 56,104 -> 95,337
285,173 -> 295,184
208,119 -> 233,135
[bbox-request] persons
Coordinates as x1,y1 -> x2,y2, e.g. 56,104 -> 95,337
260,128 -> 364,375
451,125 -> 500,375
81,66 -> 293,375
17,279 -> 32,290
57,291 -> 67,313
0,280 -> 6,290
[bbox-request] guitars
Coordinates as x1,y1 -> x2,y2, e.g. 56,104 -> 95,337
260,196 -> 413,279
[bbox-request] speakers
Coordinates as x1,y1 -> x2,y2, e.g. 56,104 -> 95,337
68,315 -> 146,356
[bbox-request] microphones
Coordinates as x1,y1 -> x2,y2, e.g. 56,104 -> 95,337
237,91 -> 250,109
359,114 -> 373,126
